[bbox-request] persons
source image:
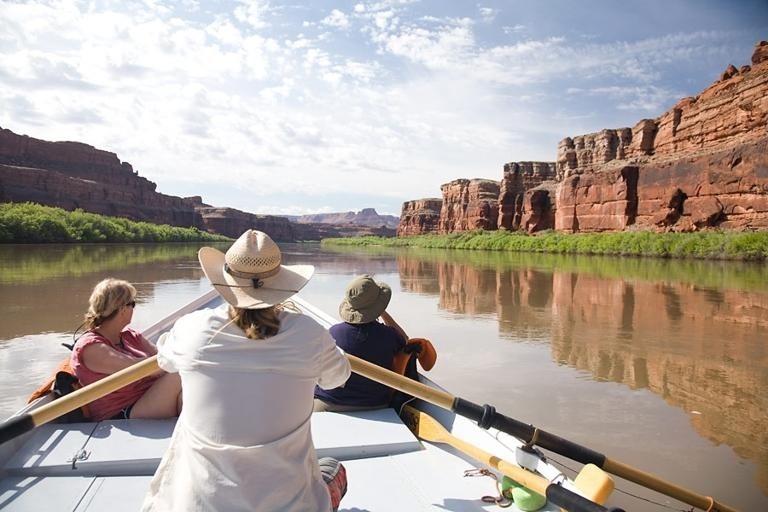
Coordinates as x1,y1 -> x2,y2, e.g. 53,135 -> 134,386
69,276 -> 182,421
313,273 -> 408,412
141,228 -> 352,512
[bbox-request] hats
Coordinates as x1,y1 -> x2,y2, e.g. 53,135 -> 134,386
198,229 -> 317,310
339,273 -> 391,327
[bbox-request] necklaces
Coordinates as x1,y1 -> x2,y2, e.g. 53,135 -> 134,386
115,342 -> 122,347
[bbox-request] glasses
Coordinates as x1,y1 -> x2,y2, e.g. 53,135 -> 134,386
120,299 -> 135,308
